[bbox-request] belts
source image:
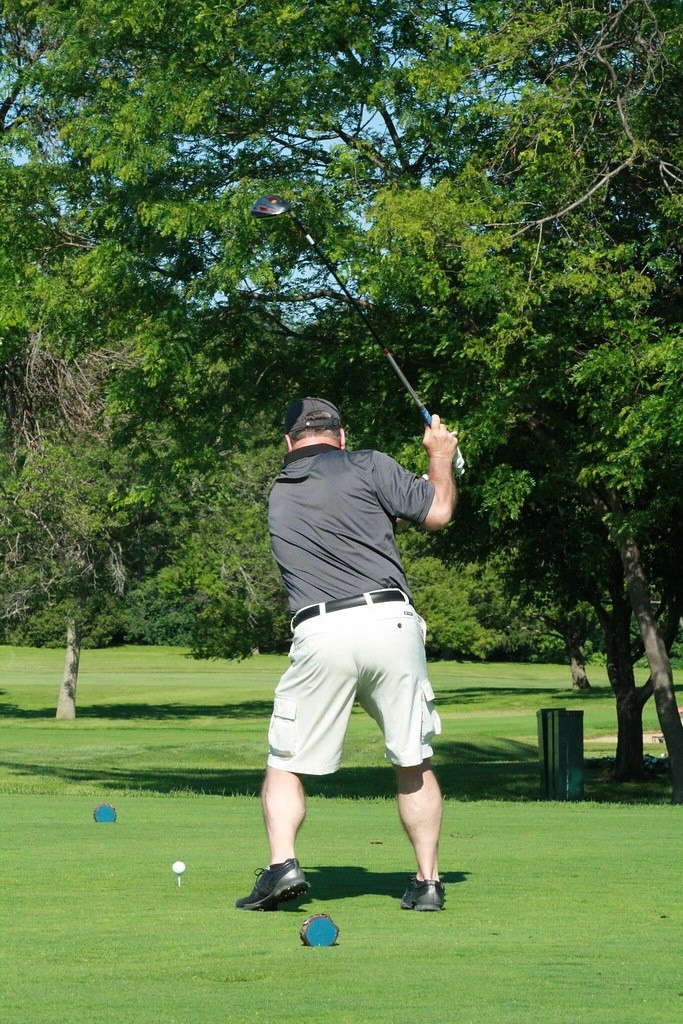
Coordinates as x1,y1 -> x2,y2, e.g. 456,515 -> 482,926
292,590 -> 405,629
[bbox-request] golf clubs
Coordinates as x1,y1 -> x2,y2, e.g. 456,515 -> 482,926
249,194 -> 466,477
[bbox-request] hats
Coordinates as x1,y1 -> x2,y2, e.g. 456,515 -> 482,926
285,397 -> 341,434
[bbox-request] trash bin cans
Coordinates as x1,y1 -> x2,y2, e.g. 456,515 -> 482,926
535,707 -> 585,800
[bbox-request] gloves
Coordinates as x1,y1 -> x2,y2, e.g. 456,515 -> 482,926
452,446 -> 466,476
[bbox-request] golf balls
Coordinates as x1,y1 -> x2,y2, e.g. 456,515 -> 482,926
171,860 -> 185,876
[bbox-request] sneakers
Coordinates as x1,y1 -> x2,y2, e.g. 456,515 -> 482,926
401,875 -> 445,912
235,858 -> 309,911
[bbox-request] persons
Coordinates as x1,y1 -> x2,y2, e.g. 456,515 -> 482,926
234,396 -> 458,910
